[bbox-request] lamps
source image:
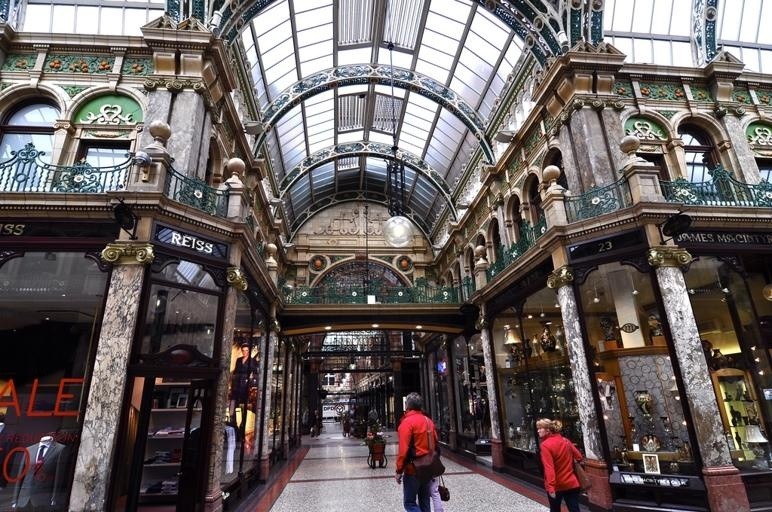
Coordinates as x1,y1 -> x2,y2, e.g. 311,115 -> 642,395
742,424 -> 772,471
455,201 -> 469,209
432,243 -> 443,252
380,2 -> 415,250
268,196 -> 284,207
242,120 -> 263,136
493,129 -> 517,144
282,242 -> 294,249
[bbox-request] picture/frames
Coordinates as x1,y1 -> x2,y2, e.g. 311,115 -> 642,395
642,453 -> 662,474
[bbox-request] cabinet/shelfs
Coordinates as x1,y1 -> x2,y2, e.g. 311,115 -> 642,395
133,377 -> 202,500
711,367 -> 772,468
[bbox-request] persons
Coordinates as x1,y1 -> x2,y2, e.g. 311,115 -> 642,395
229,344 -> 258,441
536,417 -> 584,512
471,395 -> 490,440
340,404 -> 379,439
310,410 -> 322,438
420,407 -> 445,512
11,435 -> 70,511
395,392 -> 439,512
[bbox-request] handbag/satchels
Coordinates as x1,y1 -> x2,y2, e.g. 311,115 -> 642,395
573,462 -> 593,493
407,450 -> 447,482
437,485 -> 451,502
